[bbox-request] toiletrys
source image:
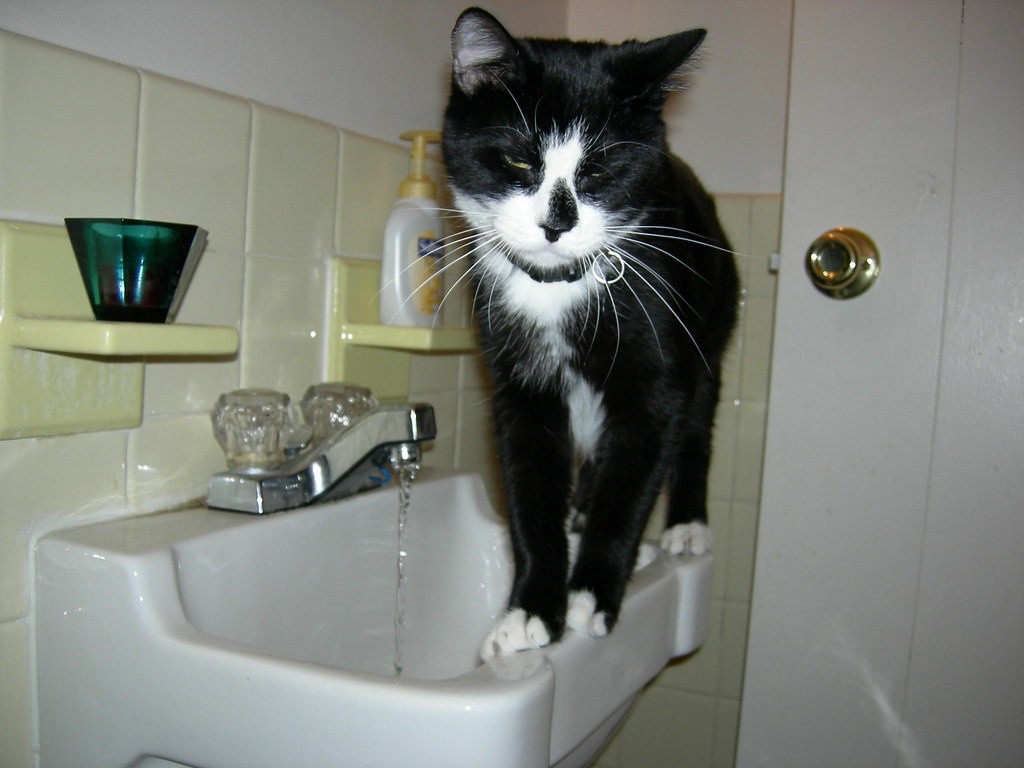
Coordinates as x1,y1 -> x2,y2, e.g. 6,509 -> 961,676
378,129 -> 446,327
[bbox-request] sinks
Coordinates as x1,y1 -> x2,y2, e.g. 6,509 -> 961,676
31,470 -> 714,768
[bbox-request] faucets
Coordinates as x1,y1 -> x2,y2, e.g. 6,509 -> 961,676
205,400 -> 438,516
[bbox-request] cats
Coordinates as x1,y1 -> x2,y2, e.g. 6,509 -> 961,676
441,8 -> 748,665
